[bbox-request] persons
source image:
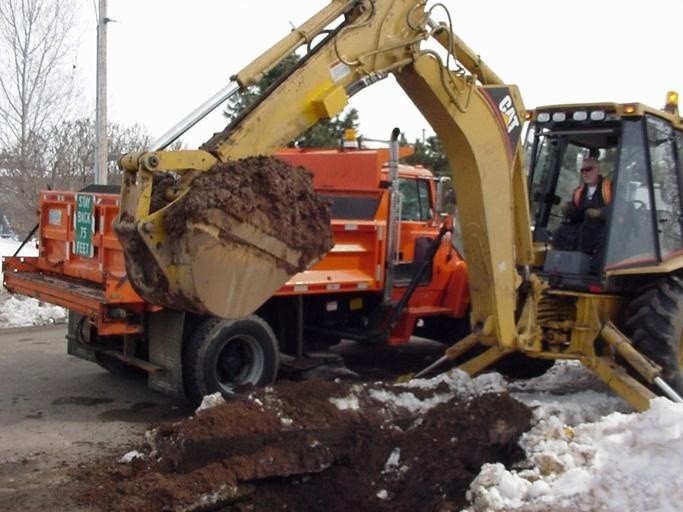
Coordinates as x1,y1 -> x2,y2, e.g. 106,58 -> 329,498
553,158 -> 614,251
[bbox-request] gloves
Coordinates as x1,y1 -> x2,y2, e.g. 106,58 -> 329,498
585,208 -> 600,218
562,201 -> 577,218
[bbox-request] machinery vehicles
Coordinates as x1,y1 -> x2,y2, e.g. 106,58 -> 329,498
112,0 -> 683,412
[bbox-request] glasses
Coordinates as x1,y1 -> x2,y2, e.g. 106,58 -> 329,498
580,166 -> 597,173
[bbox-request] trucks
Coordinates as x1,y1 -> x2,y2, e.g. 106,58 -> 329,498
0,126 -> 471,405
